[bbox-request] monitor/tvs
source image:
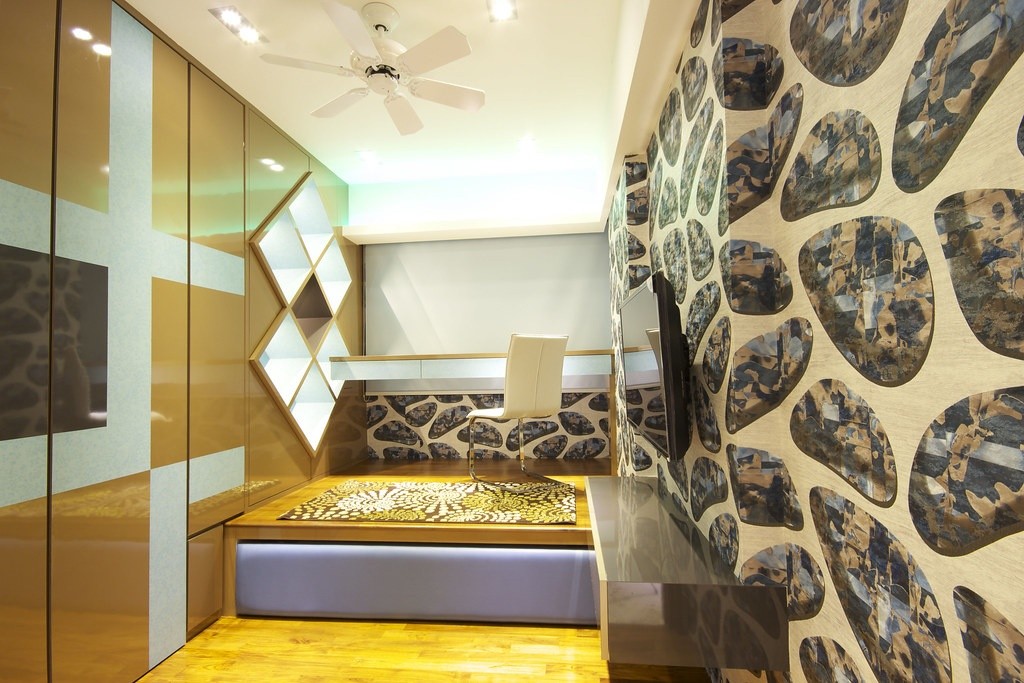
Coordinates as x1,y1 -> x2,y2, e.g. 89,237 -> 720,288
619,271 -> 691,463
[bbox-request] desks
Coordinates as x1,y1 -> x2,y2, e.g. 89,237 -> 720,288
329,350 -> 615,381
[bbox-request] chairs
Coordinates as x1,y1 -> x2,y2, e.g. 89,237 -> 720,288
466,334 -> 569,494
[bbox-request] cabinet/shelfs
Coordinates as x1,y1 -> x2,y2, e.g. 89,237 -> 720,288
585,476 -> 788,671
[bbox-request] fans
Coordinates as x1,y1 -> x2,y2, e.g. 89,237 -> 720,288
260,2 -> 485,135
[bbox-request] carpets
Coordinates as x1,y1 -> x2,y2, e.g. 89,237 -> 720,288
276,479 -> 577,525
